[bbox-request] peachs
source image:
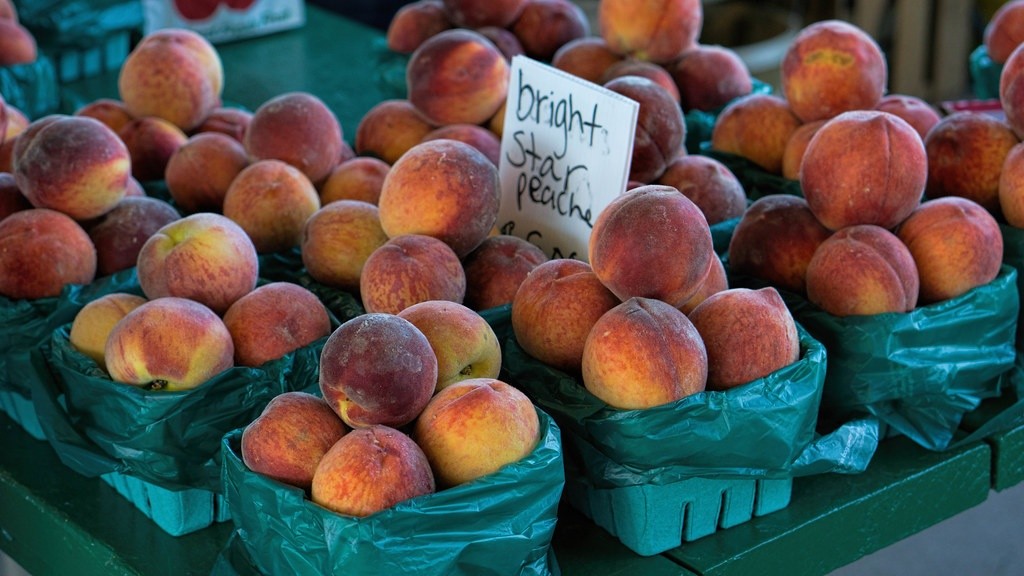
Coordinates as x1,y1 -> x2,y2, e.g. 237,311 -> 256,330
0,0 -> 1024,516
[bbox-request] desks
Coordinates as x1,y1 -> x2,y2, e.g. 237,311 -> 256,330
0,7 -> 1024,576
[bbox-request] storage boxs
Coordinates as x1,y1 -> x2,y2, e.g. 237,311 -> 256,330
0,0 -> 1018,576
